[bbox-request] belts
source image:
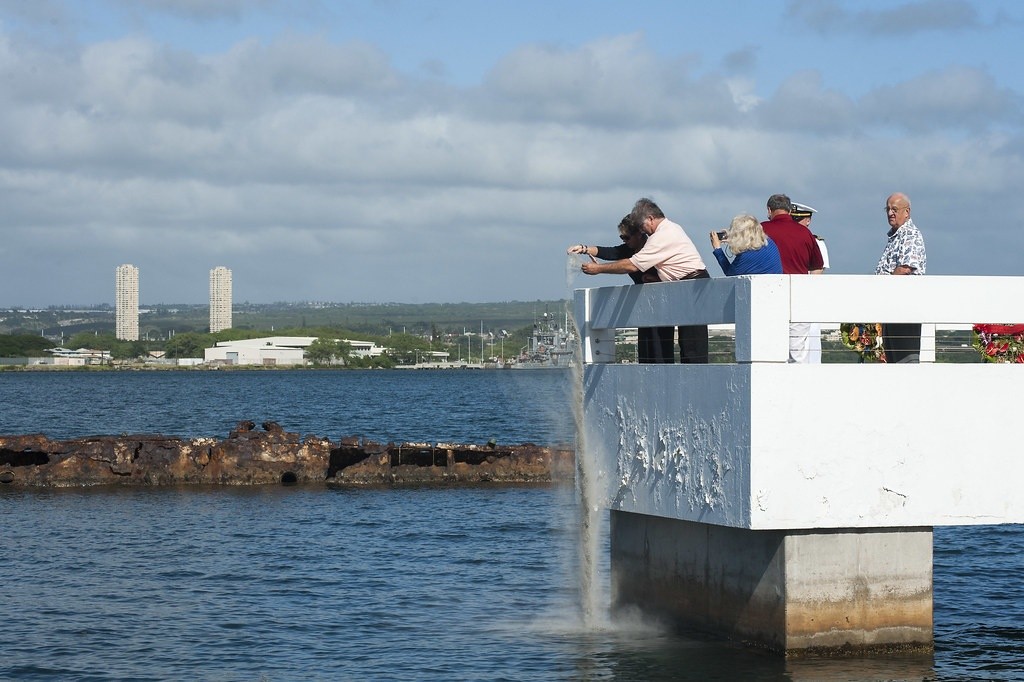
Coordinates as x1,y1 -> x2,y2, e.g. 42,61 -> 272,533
682,270 -> 704,279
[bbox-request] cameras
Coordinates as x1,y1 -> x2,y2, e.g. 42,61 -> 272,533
717,233 -> 728,240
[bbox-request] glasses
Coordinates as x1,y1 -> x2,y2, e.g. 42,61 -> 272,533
767,214 -> 770,220
619,229 -> 640,241
884,206 -> 907,212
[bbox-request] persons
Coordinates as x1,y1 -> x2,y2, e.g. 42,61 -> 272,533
581,197 -> 712,364
566,212 -> 676,365
873,191 -> 927,363
785,199 -> 830,365
708,215 -> 783,276
754,194 -> 825,365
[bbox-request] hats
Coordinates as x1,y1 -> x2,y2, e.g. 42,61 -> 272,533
791,202 -> 818,222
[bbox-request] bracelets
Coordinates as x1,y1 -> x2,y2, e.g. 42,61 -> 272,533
579,244 -> 588,255
